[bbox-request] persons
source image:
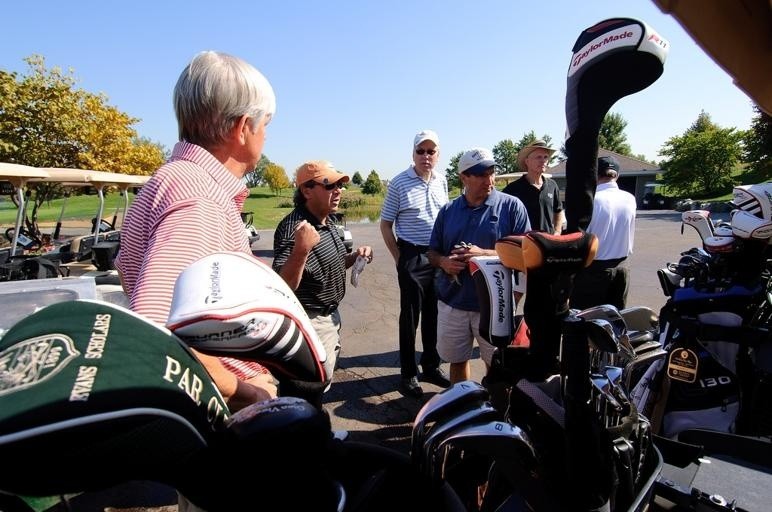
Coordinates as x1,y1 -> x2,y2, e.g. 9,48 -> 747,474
380,130 -> 450,400
501,139 -> 563,314
114,51 -> 278,417
569,156 -> 637,313
428,147 -> 531,387
272,159 -> 373,393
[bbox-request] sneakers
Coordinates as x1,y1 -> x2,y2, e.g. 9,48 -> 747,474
399,374 -> 426,402
418,365 -> 451,389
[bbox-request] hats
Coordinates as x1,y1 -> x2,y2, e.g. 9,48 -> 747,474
597,154 -> 622,181
412,128 -> 440,149
457,147 -> 503,178
293,158 -> 351,190
515,138 -> 558,172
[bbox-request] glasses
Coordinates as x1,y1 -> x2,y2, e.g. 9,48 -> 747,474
312,179 -> 345,191
413,146 -> 438,155
470,170 -> 496,179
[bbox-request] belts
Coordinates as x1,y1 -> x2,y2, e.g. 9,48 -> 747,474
303,301 -> 340,317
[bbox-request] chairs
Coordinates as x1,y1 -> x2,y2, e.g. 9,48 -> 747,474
103,229 -> 121,244
58,234 -> 104,276
